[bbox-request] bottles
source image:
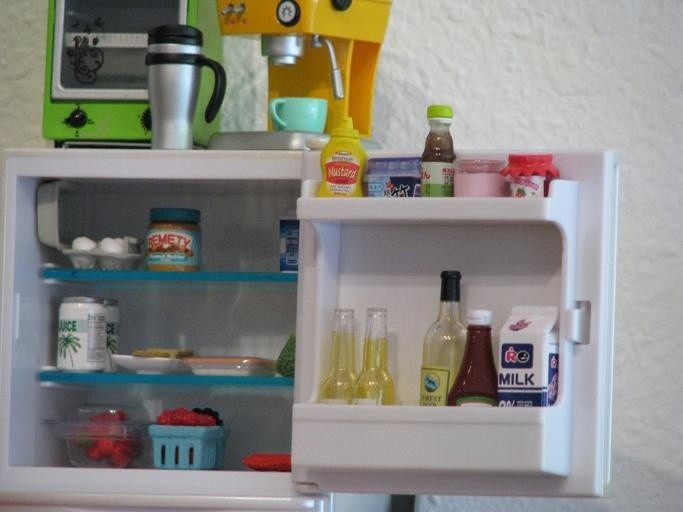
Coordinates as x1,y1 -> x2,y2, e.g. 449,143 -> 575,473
500,153 -> 562,198
420,104 -> 459,200
315,309 -> 360,405
349,306 -> 397,407
448,309 -> 502,409
419,270 -> 471,406
452,159 -> 507,197
277,197 -> 304,274
312,113 -> 366,199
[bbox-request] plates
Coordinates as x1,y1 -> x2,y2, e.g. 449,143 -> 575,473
109,353 -> 189,375
183,360 -> 279,379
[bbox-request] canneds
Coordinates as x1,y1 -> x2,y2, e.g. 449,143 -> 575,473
509,154 -> 553,197
146,206 -> 203,272
56,295 -> 120,375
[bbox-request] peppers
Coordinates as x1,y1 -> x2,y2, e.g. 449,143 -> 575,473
70,410 -> 142,469
241,453 -> 290,472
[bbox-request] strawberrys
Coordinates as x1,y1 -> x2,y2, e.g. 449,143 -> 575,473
156,407 -> 215,425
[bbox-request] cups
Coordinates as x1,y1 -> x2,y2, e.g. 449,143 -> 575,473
143,25 -> 228,149
268,96 -> 331,134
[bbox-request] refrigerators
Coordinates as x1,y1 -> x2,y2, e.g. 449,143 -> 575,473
0,134 -> 619,512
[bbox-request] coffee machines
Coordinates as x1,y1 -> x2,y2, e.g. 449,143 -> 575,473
206,0 -> 391,152
